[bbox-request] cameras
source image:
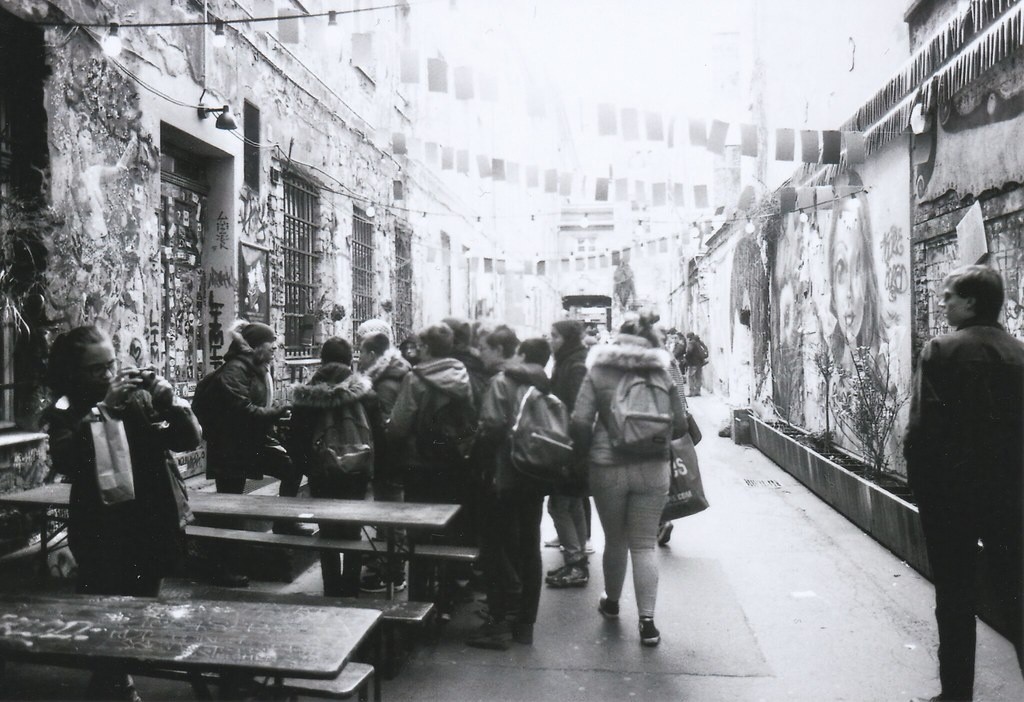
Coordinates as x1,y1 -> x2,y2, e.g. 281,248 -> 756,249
129,367 -> 156,388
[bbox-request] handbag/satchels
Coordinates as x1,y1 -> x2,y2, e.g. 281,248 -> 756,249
317,442 -> 370,484
244,427 -> 280,464
661,429 -> 709,522
90,402 -> 135,506
509,388 -> 576,482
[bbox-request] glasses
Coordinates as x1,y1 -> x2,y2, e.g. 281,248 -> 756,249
73,359 -> 122,380
943,291 -> 960,301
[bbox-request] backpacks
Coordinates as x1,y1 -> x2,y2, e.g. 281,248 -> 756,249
431,399 -> 481,472
588,366 -> 673,461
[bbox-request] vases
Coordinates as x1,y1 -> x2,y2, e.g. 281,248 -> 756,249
301,313 -> 332,344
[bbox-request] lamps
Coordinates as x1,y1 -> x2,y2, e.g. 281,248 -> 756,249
197,102 -> 238,129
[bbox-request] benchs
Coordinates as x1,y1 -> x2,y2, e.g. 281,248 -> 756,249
52,526 -> 480,702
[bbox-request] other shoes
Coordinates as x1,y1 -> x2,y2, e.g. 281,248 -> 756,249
599,595 -> 620,617
272,522 -> 315,538
87,670 -> 143,702
640,617 -> 660,643
513,623 -> 535,644
658,524 -> 673,546
212,564 -> 249,586
544,536 -> 562,547
465,620 -> 513,650
585,538 -> 596,554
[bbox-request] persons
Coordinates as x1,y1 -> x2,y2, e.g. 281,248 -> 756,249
203,323 -> 314,587
902,265 -> 1024,702
666,328 -> 704,397
289,312 -> 592,652
568,311 -> 688,645
49,325 -> 202,702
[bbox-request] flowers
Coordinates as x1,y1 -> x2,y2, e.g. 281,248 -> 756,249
302,286 -> 346,322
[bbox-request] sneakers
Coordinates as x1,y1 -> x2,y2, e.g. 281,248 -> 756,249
547,557 -> 590,586
360,574 -> 408,592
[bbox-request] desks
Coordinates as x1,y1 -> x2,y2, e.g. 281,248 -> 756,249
0,482 -> 462,680
0,585 -> 382,702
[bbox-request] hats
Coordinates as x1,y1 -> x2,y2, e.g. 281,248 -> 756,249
242,324 -> 275,347
